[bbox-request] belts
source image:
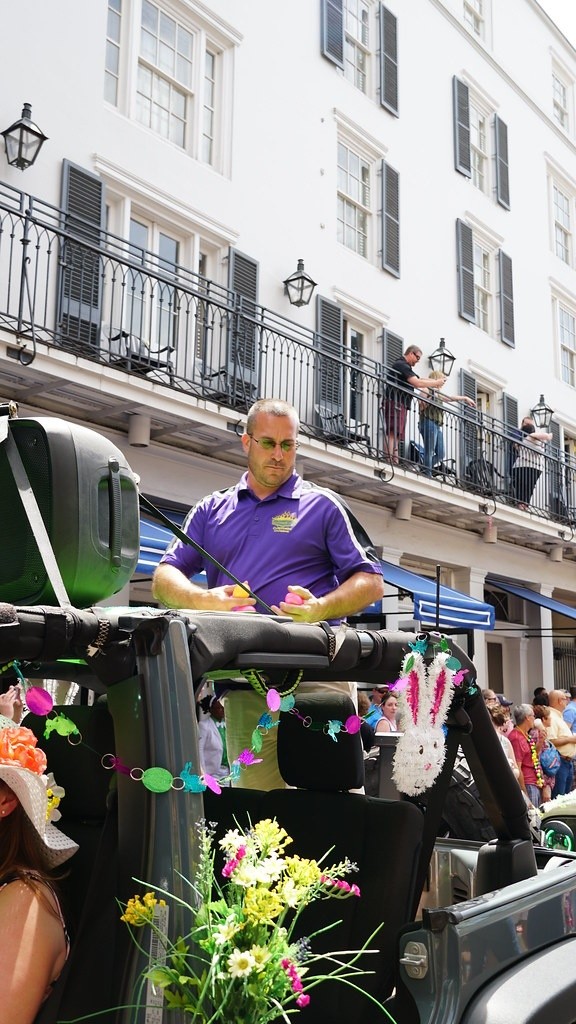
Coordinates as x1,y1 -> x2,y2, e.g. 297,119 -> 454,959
561,755 -> 573,761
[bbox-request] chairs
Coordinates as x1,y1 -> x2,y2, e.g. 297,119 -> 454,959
103,326 -> 174,386
259,687 -> 427,1024
17,703 -> 123,1024
315,405 -> 372,457
198,362 -> 261,409
468,459 -> 516,507
410,441 -> 457,484
555,498 -> 576,525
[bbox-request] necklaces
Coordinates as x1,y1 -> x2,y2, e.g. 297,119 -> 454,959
516,725 -> 544,788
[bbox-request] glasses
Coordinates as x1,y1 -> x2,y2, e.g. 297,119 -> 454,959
413,352 -> 420,360
528,715 -> 536,718
251,437 -> 300,451
377,689 -> 389,694
564,690 -> 570,693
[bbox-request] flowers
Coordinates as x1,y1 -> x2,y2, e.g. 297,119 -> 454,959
66,816 -> 397,1024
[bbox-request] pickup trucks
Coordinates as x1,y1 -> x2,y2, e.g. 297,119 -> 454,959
0,603 -> 576,1023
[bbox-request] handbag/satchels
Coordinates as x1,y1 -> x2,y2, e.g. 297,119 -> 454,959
540,742 -> 561,776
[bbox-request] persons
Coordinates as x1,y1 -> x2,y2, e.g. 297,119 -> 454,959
419,372 -> 477,474
383,346 -> 447,463
0,715 -> 69,1024
151,397 -> 384,623
480,684 -> 576,809
358,683 -> 400,732
511,416 -> 554,513
0,684 -> 25,723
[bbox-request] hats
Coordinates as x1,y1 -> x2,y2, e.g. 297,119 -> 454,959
0,714 -> 81,868
496,694 -> 514,706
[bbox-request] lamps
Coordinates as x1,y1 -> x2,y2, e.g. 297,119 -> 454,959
549,547 -> 564,562
394,497 -> 413,521
283,258 -> 318,308
428,337 -> 456,376
530,394 -> 554,429
125,413 -> 153,446
1,102 -> 48,170
484,526 -> 497,543
